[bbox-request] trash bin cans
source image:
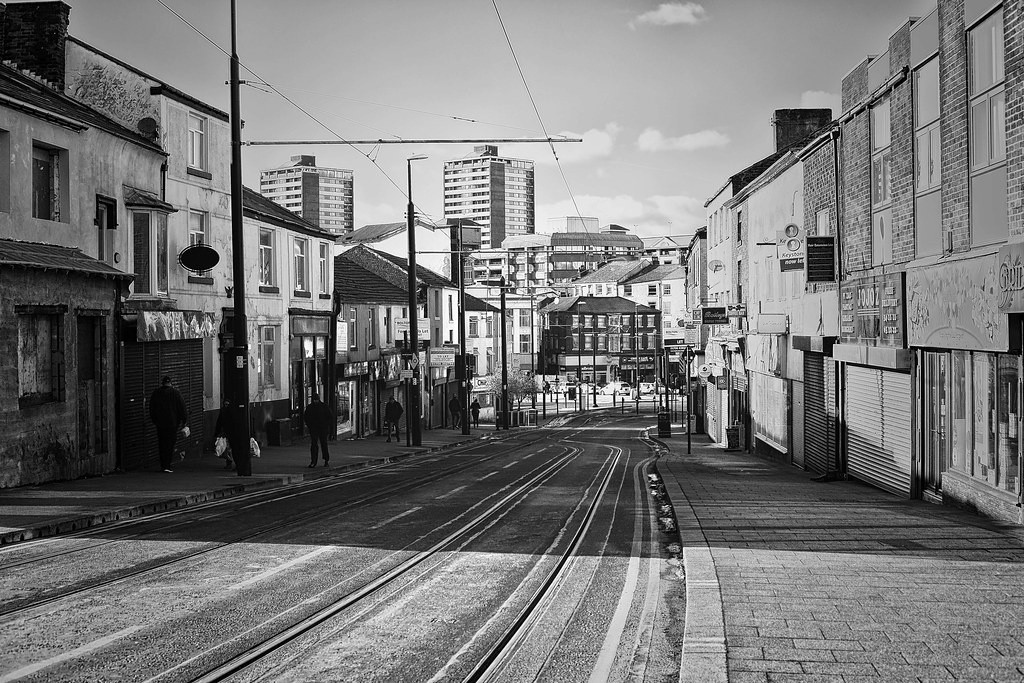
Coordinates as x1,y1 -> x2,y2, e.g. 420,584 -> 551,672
528,409 -> 537,427
725,426 -> 740,449
496,411 -> 504,431
508,410 -> 526,427
657,412 -> 672,438
568,387 -> 576,401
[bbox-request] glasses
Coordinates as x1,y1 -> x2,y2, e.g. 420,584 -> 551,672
224,403 -> 229,406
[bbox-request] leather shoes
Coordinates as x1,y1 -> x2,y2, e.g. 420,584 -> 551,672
324,462 -> 329,467
308,464 -> 316,468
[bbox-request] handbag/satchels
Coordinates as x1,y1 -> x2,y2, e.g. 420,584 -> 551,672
181,427 -> 190,438
250,438 -> 260,458
215,437 -> 227,456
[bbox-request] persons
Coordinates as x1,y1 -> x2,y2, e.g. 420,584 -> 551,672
385,396 -> 404,442
213,392 -> 252,476
304,393 -> 334,467
449,393 -> 462,430
471,399 -> 481,428
149,376 -> 189,473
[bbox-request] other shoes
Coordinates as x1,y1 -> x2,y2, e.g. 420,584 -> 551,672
456,426 -> 459,429
397,439 -> 400,441
224,465 -> 233,469
386,439 -> 391,442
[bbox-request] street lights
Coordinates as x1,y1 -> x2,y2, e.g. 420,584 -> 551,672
635,302 -> 642,400
530,283 -> 542,408
577,300 -> 586,412
406,152 -> 431,448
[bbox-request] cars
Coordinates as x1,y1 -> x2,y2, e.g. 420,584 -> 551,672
598,380 -> 631,396
551,381 -> 602,394
636,382 -> 672,395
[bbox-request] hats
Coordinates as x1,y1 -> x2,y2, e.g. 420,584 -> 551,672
162,377 -> 171,383
453,394 -> 457,397
311,393 -> 320,400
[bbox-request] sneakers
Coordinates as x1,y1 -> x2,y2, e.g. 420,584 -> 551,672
164,467 -> 173,472
